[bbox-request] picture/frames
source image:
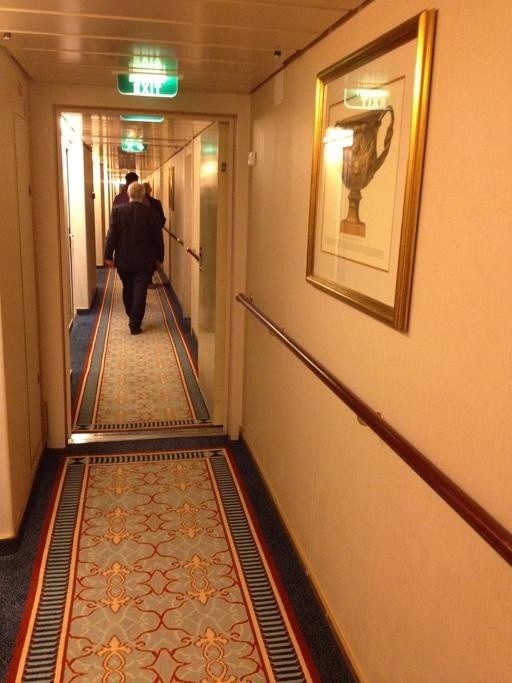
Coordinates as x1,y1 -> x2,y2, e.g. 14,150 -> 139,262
305,8 -> 438,334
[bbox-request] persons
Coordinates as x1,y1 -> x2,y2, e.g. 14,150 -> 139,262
143,182 -> 166,288
103,181 -> 163,334
113,172 -> 138,209
